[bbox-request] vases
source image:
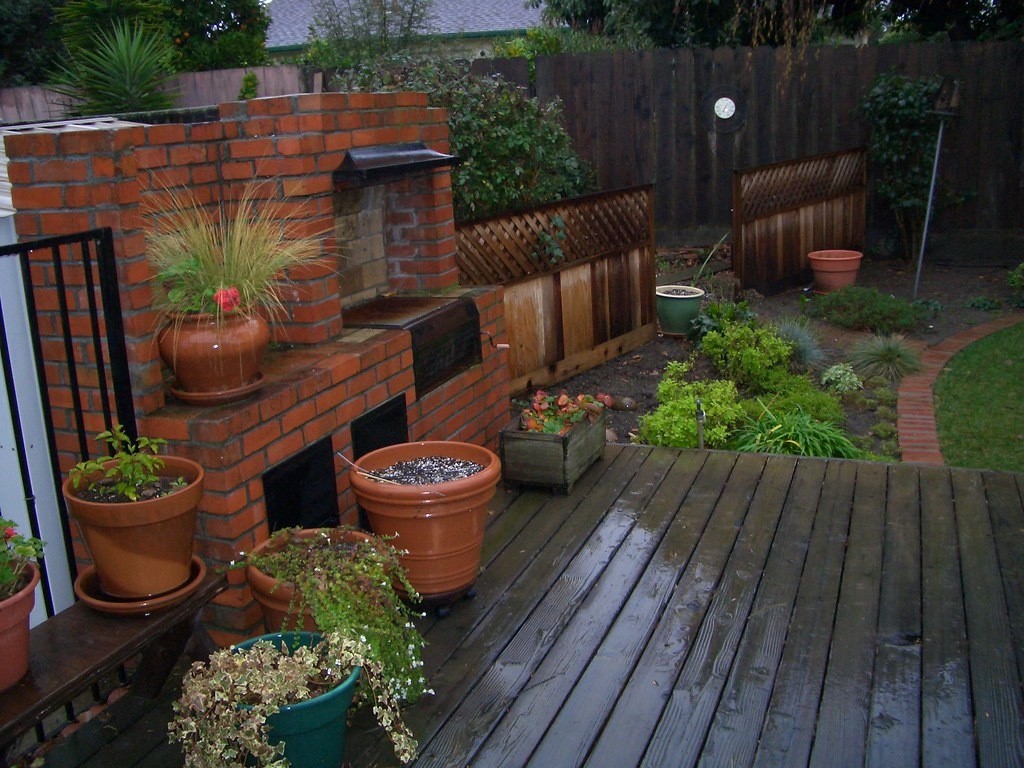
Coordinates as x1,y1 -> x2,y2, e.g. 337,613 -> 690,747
350,441 -> 502,599
247,529 -> 390,634
0,559 -> 40,691
807,250 -> 864,292
157,300 -> 271,404
656,284 -> 705,335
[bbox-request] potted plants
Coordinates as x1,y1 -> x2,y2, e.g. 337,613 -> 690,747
63,422 -> 206,617
167,630 -> 419,768
499,391 -> 613,495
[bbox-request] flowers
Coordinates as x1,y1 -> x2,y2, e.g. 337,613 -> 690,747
132,146 -> 355,380
0,516 -> 48,597
216,526 -> 436,707
